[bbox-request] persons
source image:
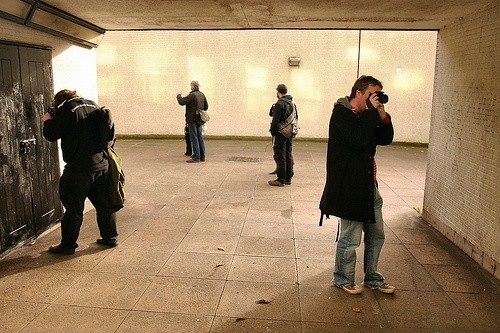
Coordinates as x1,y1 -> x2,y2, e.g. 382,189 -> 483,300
319,75 -> 395,294
177,80 -> 208,162
268,84 -> 298,186
43,89 -> 118,255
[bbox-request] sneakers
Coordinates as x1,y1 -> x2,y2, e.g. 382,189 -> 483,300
97,239 -> 118,247
49,246 -> 74,254
377,283 -> 395,294
342,282 -> 362,294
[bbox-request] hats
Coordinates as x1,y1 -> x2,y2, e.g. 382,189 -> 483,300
276,84 -> 287,93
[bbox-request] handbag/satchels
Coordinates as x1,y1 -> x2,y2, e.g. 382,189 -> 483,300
277,104 -> 300,139
195,109 -> 210,125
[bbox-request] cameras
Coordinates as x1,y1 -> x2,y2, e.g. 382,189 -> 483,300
369,91 -> 388,104
48,106 -> 57,118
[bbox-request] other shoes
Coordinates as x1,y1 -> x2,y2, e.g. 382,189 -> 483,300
269,170 -> 277,174
200,160 -> 205,162
185,153 -> 191,156
186,160 -> 199,163
285,181 -> 291,184
268,180 -> 283,186
